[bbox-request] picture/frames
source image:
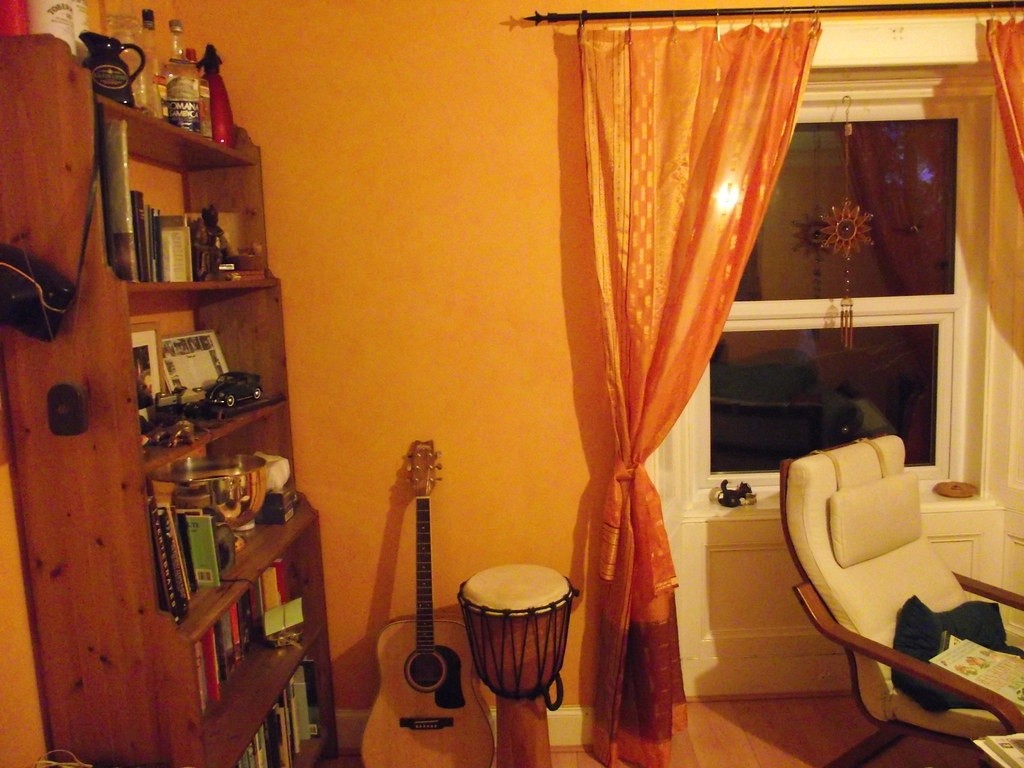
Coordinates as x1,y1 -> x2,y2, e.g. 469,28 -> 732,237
128,319 -> 230,425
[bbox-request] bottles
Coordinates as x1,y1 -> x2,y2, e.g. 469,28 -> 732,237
186,48 -> 212,140
164,20 -> 200,134
142,9 -> 170,122
202,44 -> 234,150
72,0 -> 89,44
26,0 -> 76,56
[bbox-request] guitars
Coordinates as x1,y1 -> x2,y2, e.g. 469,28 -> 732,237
359,439 -> 495,768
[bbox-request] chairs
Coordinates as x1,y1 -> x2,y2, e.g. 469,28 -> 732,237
780,433 -> 1024,768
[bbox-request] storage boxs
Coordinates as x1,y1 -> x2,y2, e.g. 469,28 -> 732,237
254,486 -> 300,526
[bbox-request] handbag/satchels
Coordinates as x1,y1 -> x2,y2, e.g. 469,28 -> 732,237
0,245 -> 76,343
47,382 -> 87,434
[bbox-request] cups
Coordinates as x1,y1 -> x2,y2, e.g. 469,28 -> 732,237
728,496 -> 739,506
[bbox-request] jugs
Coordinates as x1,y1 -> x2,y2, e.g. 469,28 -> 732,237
107,16 -> 162,120
79,31 -> 146,107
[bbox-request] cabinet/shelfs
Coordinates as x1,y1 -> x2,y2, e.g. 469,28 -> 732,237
0,33 -> 341,768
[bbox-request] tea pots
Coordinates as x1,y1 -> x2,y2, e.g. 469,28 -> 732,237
718,490 -> 740,508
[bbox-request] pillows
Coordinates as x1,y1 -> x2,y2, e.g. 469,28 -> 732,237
891,594 -> 1024,716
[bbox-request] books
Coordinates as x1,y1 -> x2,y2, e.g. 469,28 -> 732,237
96,102 -> 165,284
236,655 -> 321,768
144,481 -> 221,623
193,558 -> 288,717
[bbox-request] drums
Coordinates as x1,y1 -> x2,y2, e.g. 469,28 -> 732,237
455,561 -> 582,768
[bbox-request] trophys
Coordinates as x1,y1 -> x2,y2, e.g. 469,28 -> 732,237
169,452 -> 268,551
252,451 -> 296,524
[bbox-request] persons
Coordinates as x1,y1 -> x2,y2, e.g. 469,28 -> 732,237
194,203 -> 228,273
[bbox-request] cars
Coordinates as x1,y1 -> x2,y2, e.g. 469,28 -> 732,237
204,372 -> 263,408
